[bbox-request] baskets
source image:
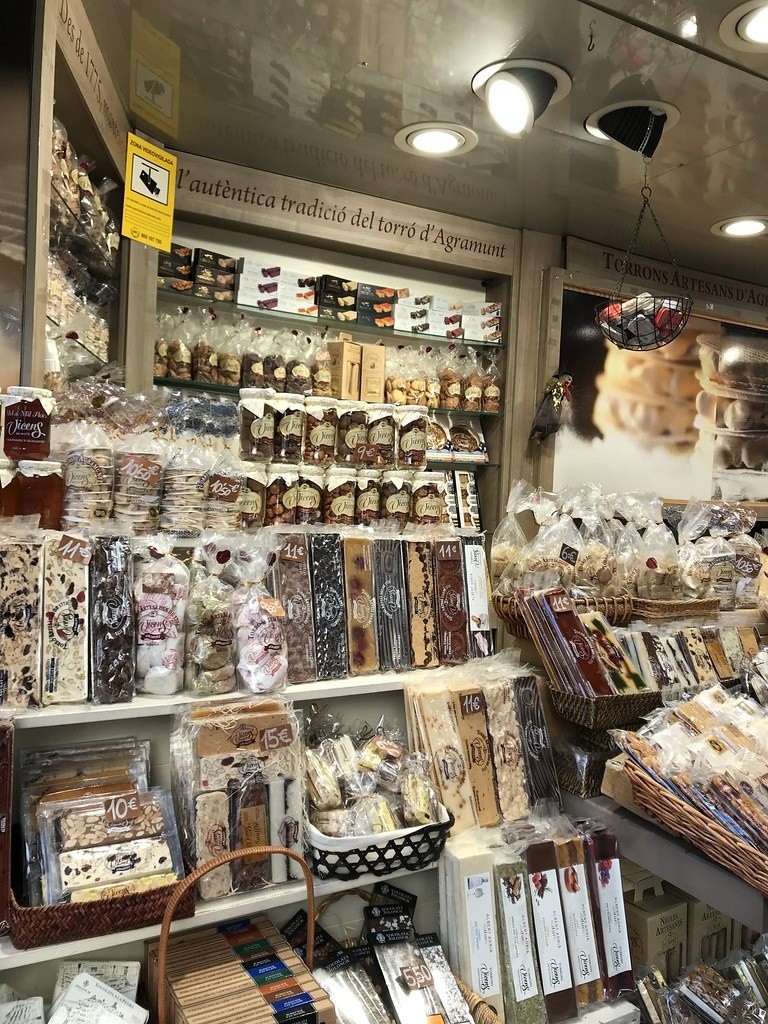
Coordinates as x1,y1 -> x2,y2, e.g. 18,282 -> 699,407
548,676 -> 752,730
492,590 -> 634,639
305,801 -> 456,882
8,845 -> 196,949
624,756 -> 767,896
633,595 -> 721,618
316,887 -> 504,1023
546,723 -> 649,800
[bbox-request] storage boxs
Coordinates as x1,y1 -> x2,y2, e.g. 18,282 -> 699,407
427,413 -> 490,463
426,469 -> 483,532
733,919 -> 760,951
623,875 -> 686,994
157,243 -> 503,346
145,912 -> 336,1024
327,332 -> 385,403
662,882 -> 731,967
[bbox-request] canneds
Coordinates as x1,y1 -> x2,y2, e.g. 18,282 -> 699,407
0,386 -> 66,531
238,387 -> 445,529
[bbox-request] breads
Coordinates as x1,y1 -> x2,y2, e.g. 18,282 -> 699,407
488,543 -> 762,611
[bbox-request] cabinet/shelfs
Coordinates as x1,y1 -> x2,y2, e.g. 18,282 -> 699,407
0,0 -> 150,403
0,536 -> 641,1024
150,137 -> 524,536
490,496 -> 768,1024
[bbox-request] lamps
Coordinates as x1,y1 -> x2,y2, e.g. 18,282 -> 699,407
710,216 -> 768,238
719,0 -> 768,54
585,100 -> 682,159
393,123 -> 479,158
471,59 -> 573,140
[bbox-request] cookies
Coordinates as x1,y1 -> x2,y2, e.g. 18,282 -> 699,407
154,340 -> 499,415
